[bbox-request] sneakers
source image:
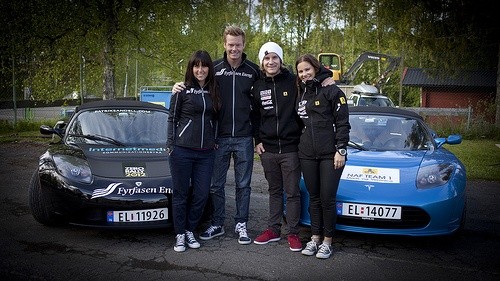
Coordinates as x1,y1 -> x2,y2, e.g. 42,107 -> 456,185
185,231 -> 200,249
302,239 -> 321,256
234,222 -> 251,245
174,233 -> 186,251
315,242 -> 333,258
199,225 -> 225,240
288,234 -> 303,252
253,229 -> 281,245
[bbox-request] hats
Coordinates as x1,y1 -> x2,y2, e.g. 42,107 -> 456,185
258,42 -> 283,68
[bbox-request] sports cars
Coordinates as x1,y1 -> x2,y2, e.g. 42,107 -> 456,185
28,102 -> 212,233
280,105 -> 468,239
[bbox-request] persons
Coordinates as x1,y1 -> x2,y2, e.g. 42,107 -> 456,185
249,42 -> 336,252
165,50 -> 223,252
295,54 -> 351,258
172,25 -> 262,244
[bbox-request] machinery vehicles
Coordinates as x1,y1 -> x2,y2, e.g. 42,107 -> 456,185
317,51 -> 403,99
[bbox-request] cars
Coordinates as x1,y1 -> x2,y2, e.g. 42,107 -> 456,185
346,84 -> 396,108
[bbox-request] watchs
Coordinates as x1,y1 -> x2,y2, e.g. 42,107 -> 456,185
337,149 -> 347,156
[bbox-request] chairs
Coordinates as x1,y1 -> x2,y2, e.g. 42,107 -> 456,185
373,119 -> 410,148
349,118 -> 371,143
